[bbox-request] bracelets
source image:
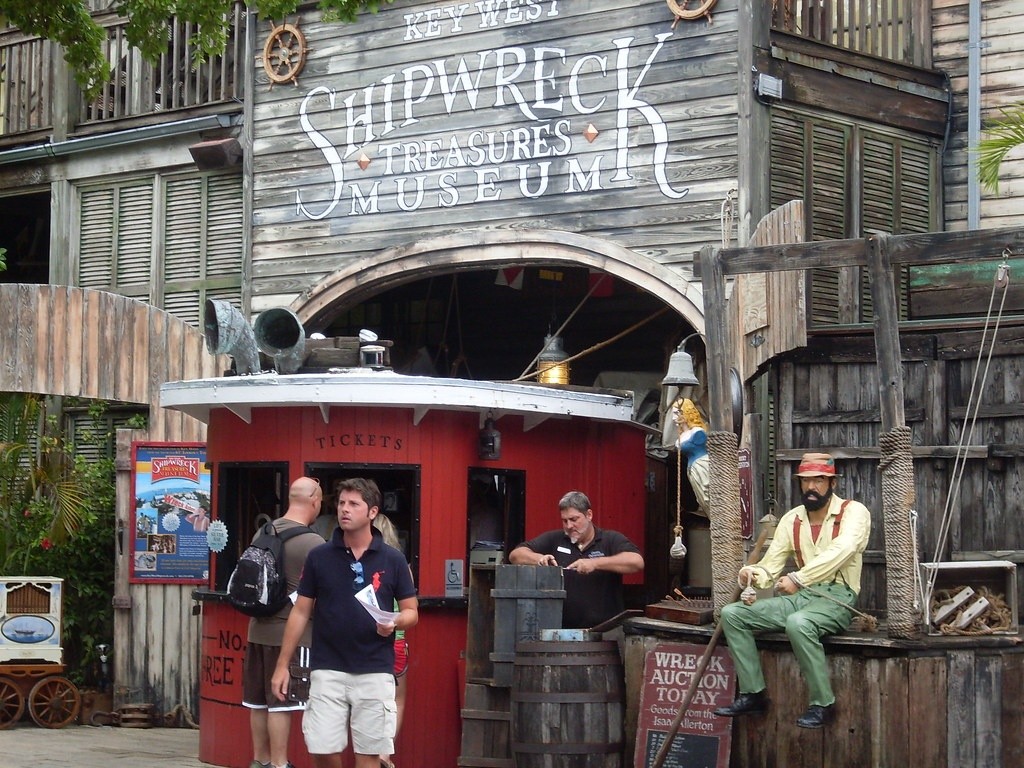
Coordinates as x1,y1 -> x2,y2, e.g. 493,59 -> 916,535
394,620 -> 397,629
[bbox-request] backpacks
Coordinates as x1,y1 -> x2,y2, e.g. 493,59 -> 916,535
226,520 -> 316,617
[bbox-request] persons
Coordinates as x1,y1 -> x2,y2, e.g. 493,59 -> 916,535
712,453 -> 871,729
271,478 -> 419,768
671,398 -> 710,521
241,477 -> 327,768
138,513 -> 149,532
509,491 -> 644,629
186,506 -> 210,532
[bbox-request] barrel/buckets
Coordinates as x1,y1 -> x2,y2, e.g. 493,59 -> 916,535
511,640 -> 629,768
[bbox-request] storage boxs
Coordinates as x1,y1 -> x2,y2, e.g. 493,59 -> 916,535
644,603 -> 714,625
539,627 -> 602,642
462,565 -> 566,688
920,562 -> 1018,636
457,683 -> 514,768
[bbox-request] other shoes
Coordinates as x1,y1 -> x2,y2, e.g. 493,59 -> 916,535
249,760 -> 295,768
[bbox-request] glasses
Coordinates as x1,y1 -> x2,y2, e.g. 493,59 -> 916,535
309,478 -> 320,498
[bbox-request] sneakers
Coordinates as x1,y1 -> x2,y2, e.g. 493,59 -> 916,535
796,705 -> 835,728
713,692 -> 770,717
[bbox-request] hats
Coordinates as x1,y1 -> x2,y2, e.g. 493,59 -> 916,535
793,453 -> 843,477
367,479 -> 381,497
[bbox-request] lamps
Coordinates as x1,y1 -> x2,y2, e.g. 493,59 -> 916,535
536,268 -> 571,382
186,139 -> 243,173
